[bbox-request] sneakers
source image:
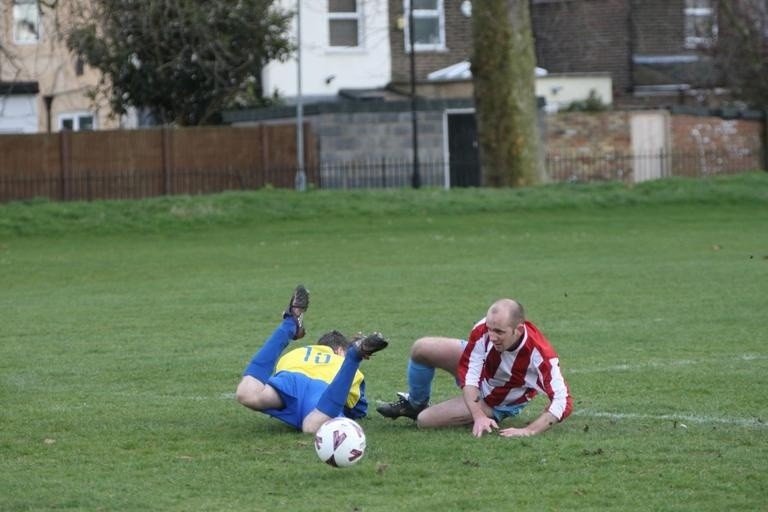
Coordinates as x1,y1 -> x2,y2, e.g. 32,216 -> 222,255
349,332 -> 388,360
376,391 -> 430,420
288,284 -> 309,340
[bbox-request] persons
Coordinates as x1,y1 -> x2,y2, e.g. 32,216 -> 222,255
374,294 -> 576,442
232,279 -> 390,440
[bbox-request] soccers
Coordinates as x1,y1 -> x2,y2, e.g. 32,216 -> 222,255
315,417 -> 366,470
